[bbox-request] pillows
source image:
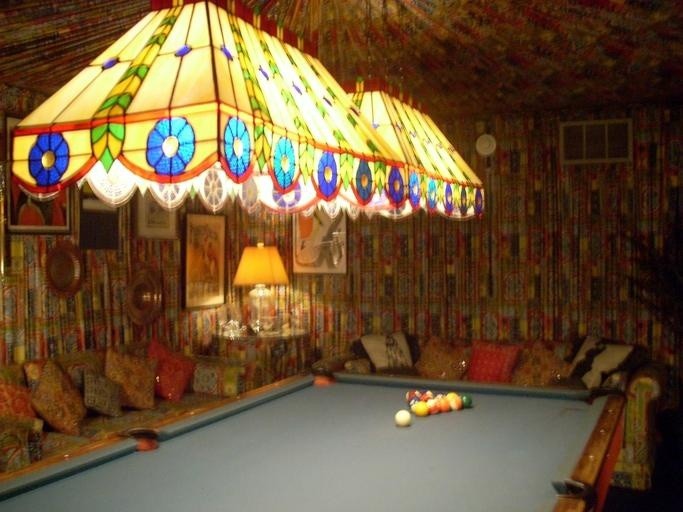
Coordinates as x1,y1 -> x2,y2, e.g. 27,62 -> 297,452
0,338 -> 197,438
353,329 -> 637,390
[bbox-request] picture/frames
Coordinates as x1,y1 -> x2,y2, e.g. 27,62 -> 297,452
3,113 -> 72,236
181,210 -> 227,311
129,190 -> 181,242
290,213 -> 349,276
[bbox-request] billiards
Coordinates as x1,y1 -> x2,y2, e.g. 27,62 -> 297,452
405,389 -> 472,416
395,409 -> 411,427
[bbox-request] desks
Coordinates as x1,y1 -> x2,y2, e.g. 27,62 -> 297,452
0,368 -> 629,512
219,326 -> 308,392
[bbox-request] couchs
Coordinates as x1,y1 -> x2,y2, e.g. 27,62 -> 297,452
312,328 -> 669,495
0,335 -> 263,483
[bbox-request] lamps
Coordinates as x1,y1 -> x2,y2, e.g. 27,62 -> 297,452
231,241 -> 290,332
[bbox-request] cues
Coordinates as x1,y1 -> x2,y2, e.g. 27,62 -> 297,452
593,400 -> 625,511
